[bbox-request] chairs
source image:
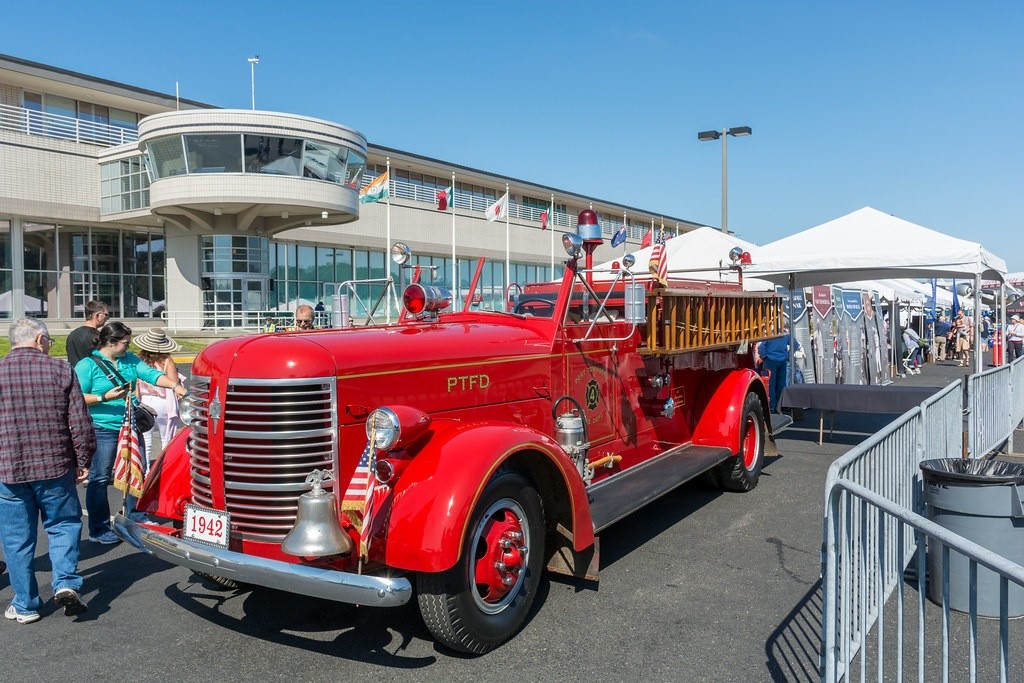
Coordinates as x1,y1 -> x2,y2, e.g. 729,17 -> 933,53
903,347 -> 916,375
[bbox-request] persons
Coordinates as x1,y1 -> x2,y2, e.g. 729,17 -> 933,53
0,317 -> 97,623
285,305 -> 323,331
246,147 -> 266,172
264,316 -> 278,332
349,316 -> 353,327
758,328 -> 805,413
924,310 -> 1023,365
73,322 -> 187,544
315,302 -> 327,324
66,300 -> 109,367
133,327 -> 183,477
899,326 -> 922,368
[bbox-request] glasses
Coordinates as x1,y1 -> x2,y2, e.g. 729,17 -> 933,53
35,335 -> 56,347
111,338 -> 129,347
97,313 -> 108,318
297,320 -> 310,324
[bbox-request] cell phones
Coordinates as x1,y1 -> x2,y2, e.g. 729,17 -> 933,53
119,381 -> 134,392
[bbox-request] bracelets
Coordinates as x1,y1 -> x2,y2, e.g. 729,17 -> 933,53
102,394 -> 107,402
173,383 -> 179,391
99,395 -> 102,402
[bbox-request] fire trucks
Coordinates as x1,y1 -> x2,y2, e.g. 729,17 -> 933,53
110,206 -> 796,655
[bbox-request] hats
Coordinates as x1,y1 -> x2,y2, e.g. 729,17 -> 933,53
1010,315 -> 1019,323
133,328 -> 177,353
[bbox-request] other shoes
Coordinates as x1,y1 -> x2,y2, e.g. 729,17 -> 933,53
957,363 -> 964,367
966,360 -> 969,366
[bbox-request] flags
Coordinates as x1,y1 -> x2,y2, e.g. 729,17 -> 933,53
640,227 -> 652,249
485,193 -> 507,222
540,206 -> 552,230
342,439 -> 391,560
649,226 -> 668,288
360,172 -> 389,203
113,400 -> 144,498
611,225 -> 626,248
437,186 -> 454,211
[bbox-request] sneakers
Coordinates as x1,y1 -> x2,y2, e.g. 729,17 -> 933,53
54,589 -> 87,617
89,530 -> 120,544
3,603 -> 40,624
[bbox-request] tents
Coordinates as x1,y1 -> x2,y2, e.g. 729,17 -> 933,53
991,295 -> 1023,321
554,207 -> 1008,374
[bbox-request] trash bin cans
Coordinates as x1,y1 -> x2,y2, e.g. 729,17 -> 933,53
919,457 -> 1024,621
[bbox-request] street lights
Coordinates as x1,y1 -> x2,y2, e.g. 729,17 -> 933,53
696,124 -> 754,235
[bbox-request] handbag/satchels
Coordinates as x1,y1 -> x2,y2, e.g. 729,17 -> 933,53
917,338 -> 930,347
134,402 -> 157,432
